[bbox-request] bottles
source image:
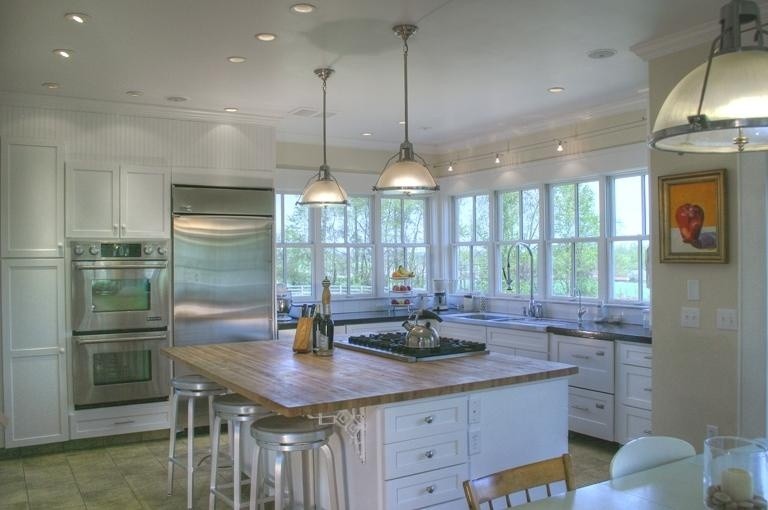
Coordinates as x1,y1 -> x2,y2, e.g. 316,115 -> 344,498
320,304 -> 333,351
312,307 -> 323,353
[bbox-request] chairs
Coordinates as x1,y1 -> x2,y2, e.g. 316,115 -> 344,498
464,454 -> 577,510
609,436 -> 696,480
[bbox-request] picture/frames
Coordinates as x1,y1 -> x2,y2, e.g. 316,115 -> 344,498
658,168 -> 727,264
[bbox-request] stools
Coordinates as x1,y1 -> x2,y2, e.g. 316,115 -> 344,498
209,393 -> 274,510
249,415 -> 339,510
167,374 -> 228,509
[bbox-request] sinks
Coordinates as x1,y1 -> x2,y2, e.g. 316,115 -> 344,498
448,311 -> 519,321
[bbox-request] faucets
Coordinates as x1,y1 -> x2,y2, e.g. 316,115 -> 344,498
568,287 -> 591,326
502,241 -> 535,318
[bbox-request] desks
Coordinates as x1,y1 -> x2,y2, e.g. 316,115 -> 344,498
159,332 -> 580,510
502,442 -> 767,509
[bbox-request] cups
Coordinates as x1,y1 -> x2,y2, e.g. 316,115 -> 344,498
703,435 -> 768,510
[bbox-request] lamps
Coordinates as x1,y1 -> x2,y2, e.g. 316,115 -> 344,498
374,24 -> 440,194
647,1 -> 764,155
295,68 -> 350,207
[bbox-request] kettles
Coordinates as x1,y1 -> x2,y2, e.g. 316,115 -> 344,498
401,309 -> 444,354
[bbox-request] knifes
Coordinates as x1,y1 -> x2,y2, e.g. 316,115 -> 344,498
310,304 -> 315,317
302,304 -> 308,317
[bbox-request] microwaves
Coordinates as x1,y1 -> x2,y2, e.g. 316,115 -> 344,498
72,331 -> 171,411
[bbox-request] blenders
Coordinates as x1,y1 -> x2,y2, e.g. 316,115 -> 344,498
432,280 -> 449,311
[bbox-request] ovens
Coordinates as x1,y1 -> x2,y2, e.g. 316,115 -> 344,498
72,237 -> 169,335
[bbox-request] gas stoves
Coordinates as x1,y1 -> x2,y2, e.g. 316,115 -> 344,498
333,332 -> 490,363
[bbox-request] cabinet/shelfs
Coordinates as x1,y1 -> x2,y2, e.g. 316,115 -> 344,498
278,325 -> 345,339
613,340 -> 652,445
436,321 -> 485,346
554,335 -> 613,442
0,136 -> 67,450
345,320 -> 425,340
64,160 -> 173,240
382,395 -> 469,510
486,326 -> 548,365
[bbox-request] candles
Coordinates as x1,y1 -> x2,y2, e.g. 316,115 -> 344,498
720,464 -> 753,501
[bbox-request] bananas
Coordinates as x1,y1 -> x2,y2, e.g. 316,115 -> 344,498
398,266 -> 413,277
